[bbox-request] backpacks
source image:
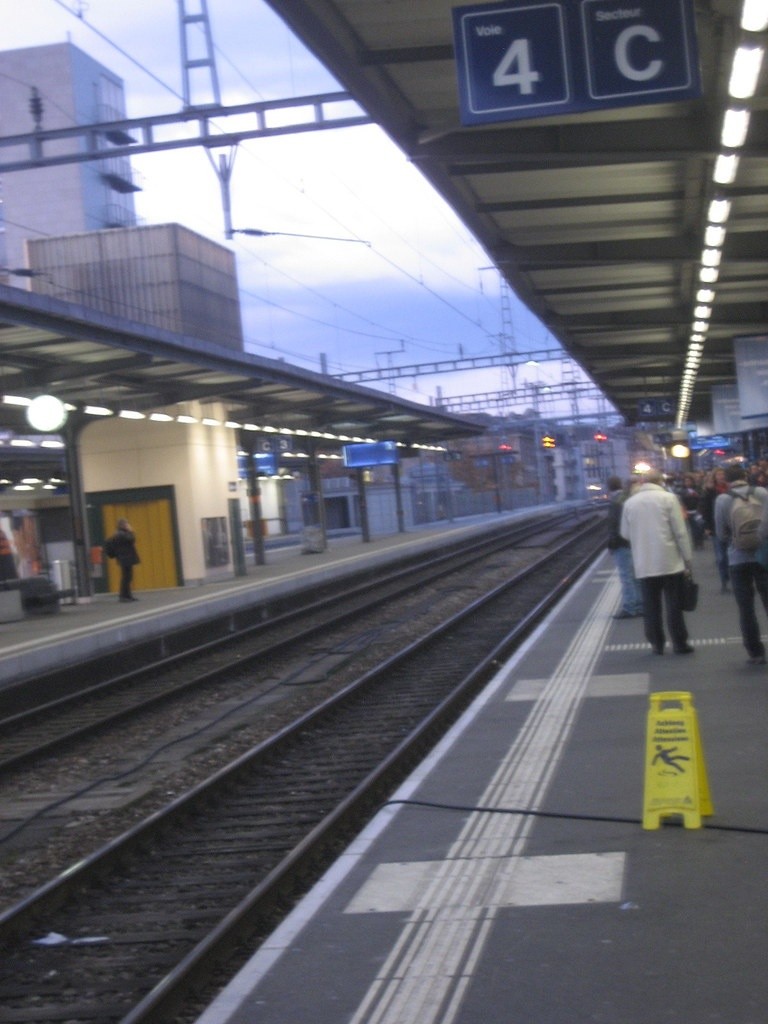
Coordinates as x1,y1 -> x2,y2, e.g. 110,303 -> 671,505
724,484 -> 766,553
104,538 -> 118,557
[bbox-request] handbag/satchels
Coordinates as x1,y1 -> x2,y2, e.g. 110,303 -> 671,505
678,567 -> 699,612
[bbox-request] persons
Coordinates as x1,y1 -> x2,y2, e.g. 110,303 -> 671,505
714,464 -> 768,664
606,476 -> 645,618
112,519 -> 141,602
618,469 -> 694,656
631,459 -> 768,595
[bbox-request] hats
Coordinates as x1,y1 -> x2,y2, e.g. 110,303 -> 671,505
726,465 -> 745,482
608,476 -> 622,490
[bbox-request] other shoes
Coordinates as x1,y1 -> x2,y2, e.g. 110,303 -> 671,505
119,596 -> 136,602
672,640 -> 694,653
613,610 -> 632,618
652,643 -> 664,654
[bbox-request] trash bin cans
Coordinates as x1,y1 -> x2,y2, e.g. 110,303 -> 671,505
51,560 -> 77,606
